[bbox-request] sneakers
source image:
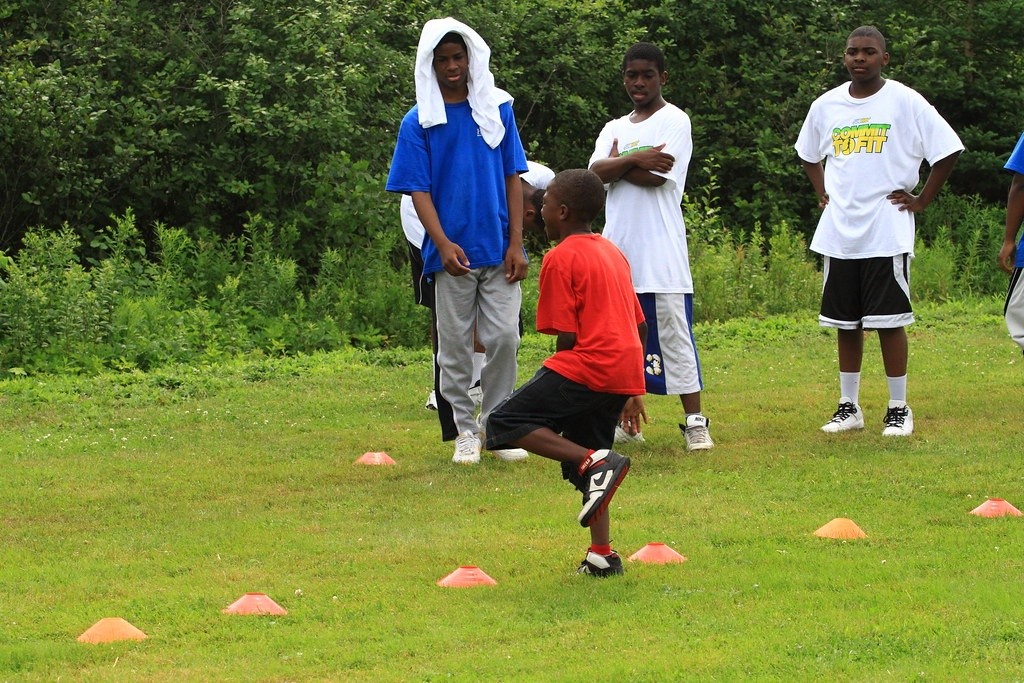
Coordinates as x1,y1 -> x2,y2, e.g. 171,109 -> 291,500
615,421 -> 644,444
468,387 -> 482,404
491,448 -> 529,461
678,414 -> 714,450
426,389 -> 438,410
568,551 -> 623,579
451,431 -> 482,464
578,448 -> 631,527
819,396 -> 864,434
881,400 -> 914,437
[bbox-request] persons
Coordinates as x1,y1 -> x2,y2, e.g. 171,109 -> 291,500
793,25 -> 965,436
999,131 -> 1023,352
383,18 -> 556,463
590,43 -> 717,451
400,161 -> 557,411
485,169 -> 648,578
